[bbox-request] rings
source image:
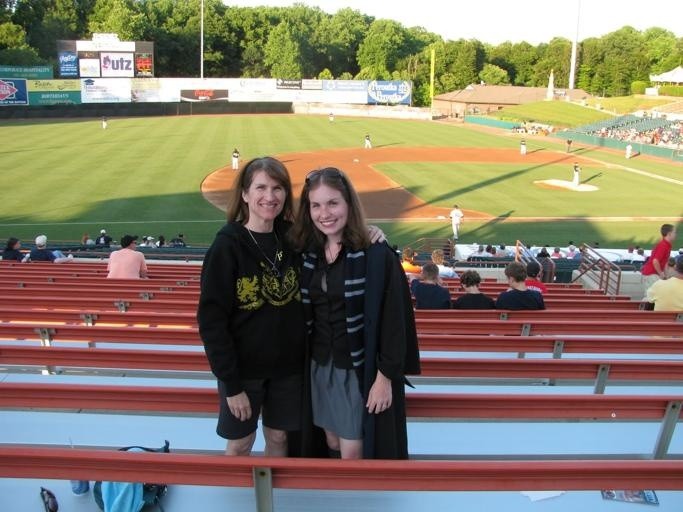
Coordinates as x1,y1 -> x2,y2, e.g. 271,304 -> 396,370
382,405 -> 386,409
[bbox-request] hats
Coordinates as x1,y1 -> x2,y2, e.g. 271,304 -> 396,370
35,235 -> 47,245
100,230 -> 105,233
527,262 -> 540,274
121,235 -> 138,248
569,245 -> 575,251
147,237 -> 154,240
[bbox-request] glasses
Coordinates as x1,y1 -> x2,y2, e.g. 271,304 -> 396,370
132,241 -> 136,244
306,168 -> 343,181
40,487 -> 58,512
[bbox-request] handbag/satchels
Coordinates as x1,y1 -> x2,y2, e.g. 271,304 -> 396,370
94,440 -> 169,512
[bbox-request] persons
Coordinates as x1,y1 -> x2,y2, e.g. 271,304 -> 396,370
232,149 -> 240,170
566,139 -> 572,153
365,134 -> 371,149
520,137 -> 526,154
102,116 -> 106,129
196,157 -> 386,457
285,168 -> 420,460
449,204 -> 463,240
574,163 -> 581,185
598,122 -> 683,159
329,112 -> 334,123
0,229 -> 187,280
393,224 -> 683,311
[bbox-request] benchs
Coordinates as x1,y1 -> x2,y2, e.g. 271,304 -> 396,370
558,112 -> 683,162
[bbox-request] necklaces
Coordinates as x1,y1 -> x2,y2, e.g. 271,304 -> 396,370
246,225 -> 279,276
328,245 -> 340,262
